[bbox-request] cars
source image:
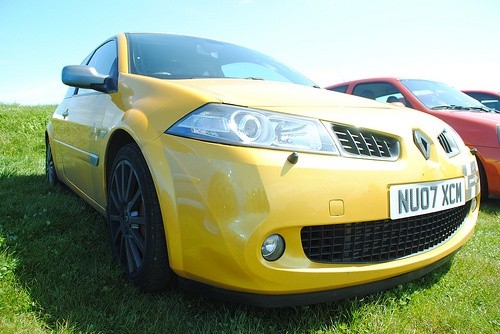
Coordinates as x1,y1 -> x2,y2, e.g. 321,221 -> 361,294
323,77 -> 500,204
44,32 -> 481,308
460,89 -> 500,114
